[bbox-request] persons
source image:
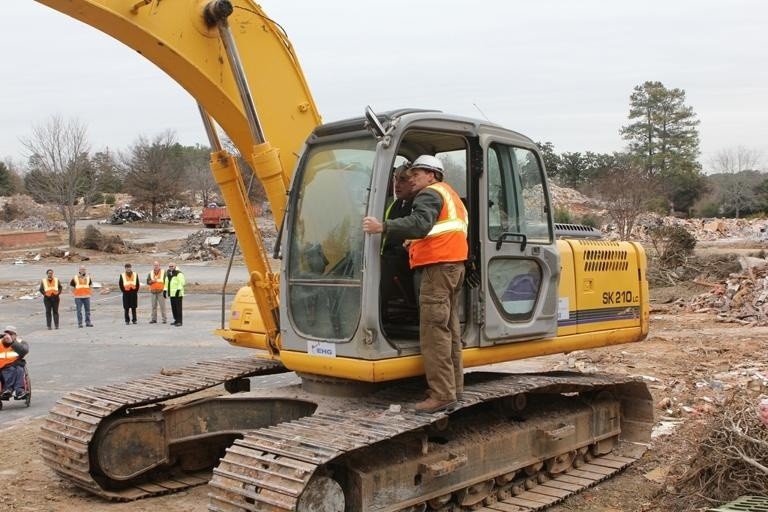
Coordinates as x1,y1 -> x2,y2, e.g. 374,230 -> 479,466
361,156 -> 473,412
325,162 -> 419,325
0,324 -> 31,400
163,262 -> 185,326
69,264 -> 94,328
118,263 -> 140,325
146,261 -> 169,324
39,269 -> 63,329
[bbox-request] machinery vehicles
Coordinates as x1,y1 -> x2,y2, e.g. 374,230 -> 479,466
37,0 -> 655,512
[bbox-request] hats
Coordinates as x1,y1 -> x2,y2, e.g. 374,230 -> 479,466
4,325 -> 17,336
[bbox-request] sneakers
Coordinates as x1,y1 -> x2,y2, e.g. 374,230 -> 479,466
78,323 -> 93,328
1,390 -> 12,398
171,322 -> 182,326
126,321 -> 137,325
17,390 -> 29,400
149,320 -> 157,323
415,392 -> 464,414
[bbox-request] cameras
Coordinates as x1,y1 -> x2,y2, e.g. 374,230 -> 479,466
0,333 -> 6,339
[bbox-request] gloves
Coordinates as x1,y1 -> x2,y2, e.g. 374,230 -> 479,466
176,290 -> 179,297
464,269 -> 481,289
163,291 -> 166,298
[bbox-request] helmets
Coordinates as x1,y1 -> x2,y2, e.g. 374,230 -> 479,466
410,154 -> 444,176
